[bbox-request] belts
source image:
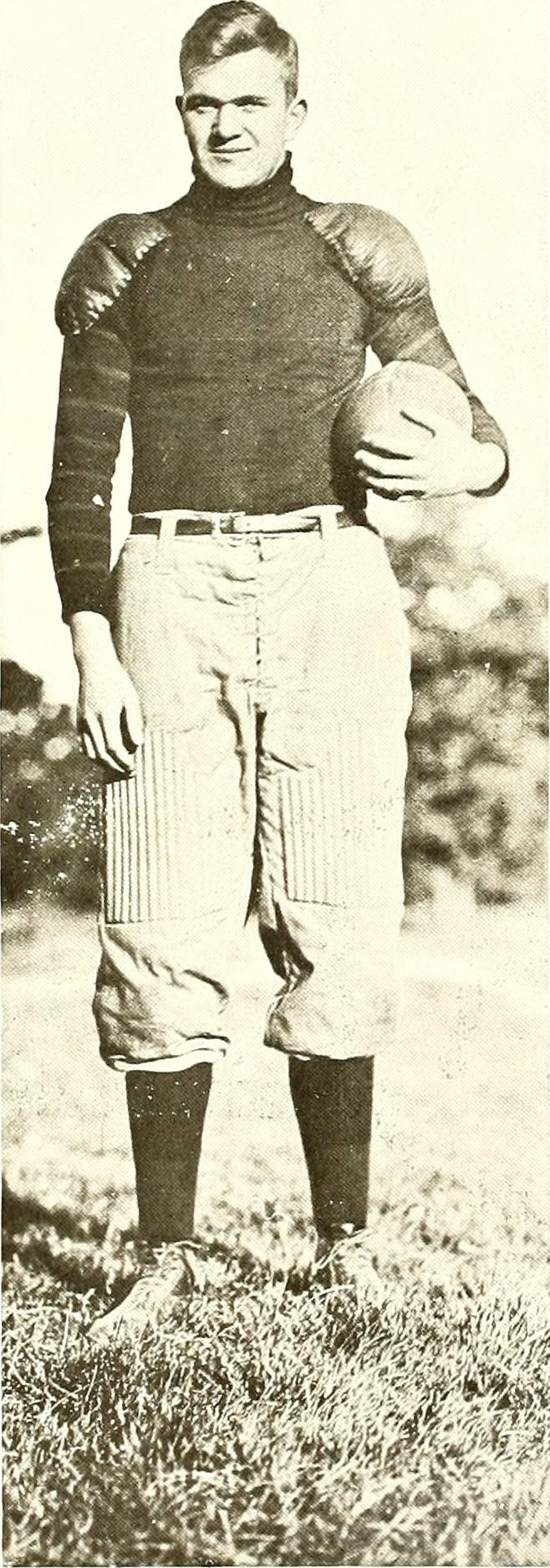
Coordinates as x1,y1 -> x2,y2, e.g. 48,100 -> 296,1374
129,511 -> 372,539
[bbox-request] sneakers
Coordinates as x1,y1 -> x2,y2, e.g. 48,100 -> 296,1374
82,1243 -> 201,1346
309,1234 -> 389,1312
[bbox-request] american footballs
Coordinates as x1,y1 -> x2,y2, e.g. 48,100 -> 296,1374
335,359 -> 472,488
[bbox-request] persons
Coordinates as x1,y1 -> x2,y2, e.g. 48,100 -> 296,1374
44,0 -> 512,1351
